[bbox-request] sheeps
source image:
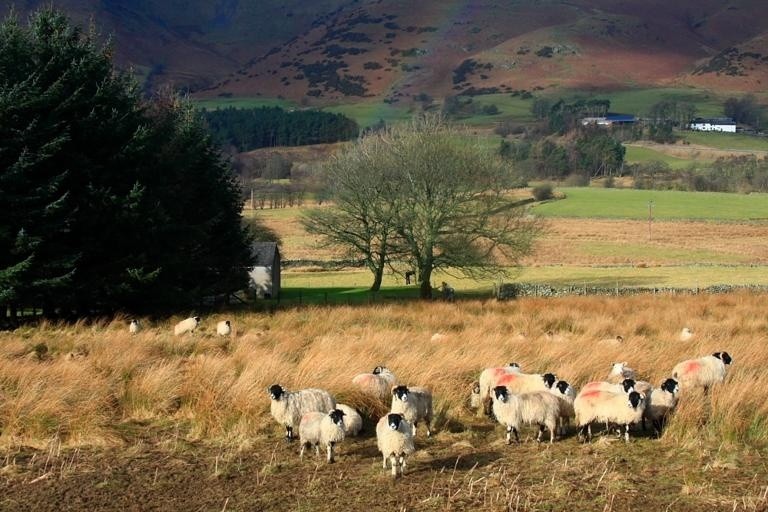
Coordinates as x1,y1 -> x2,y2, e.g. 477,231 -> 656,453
265,365 -> 434,480
469,361 -> 679,446
175,316 -> 200,336
129,318 -> 141,335
671,351 -> 733,394
217,320 -> 232,337
681,327 -> 694,342
